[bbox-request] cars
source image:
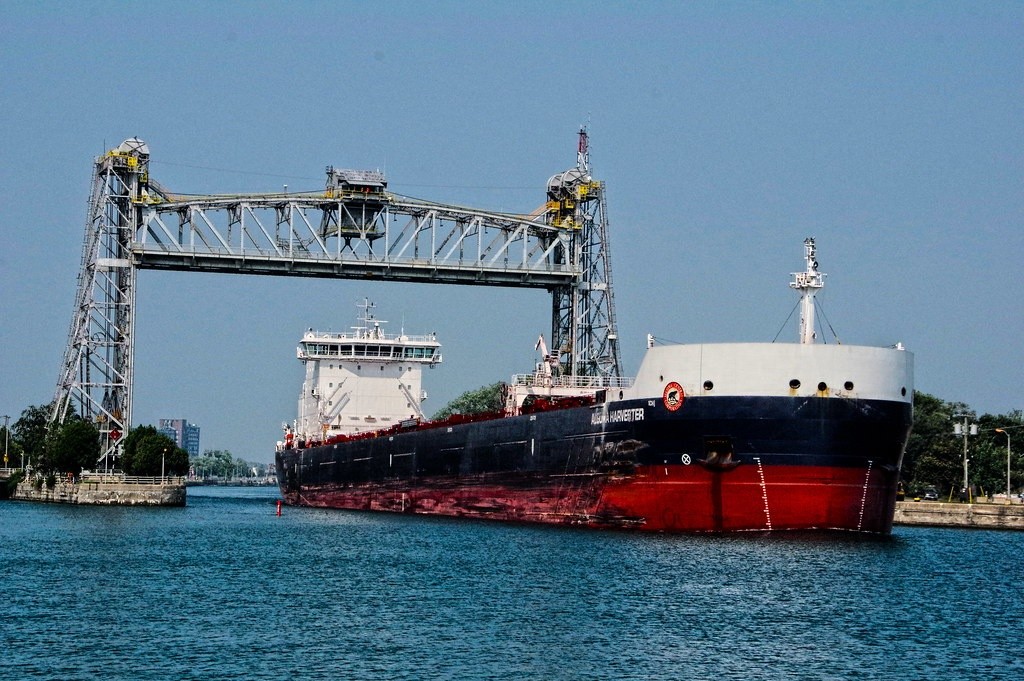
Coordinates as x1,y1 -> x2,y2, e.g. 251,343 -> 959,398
918,488 -> 939,501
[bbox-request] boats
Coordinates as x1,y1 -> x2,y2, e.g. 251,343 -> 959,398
274,235 -> 914,536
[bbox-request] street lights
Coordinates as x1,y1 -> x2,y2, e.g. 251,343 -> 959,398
996,429 -> 1011,497
